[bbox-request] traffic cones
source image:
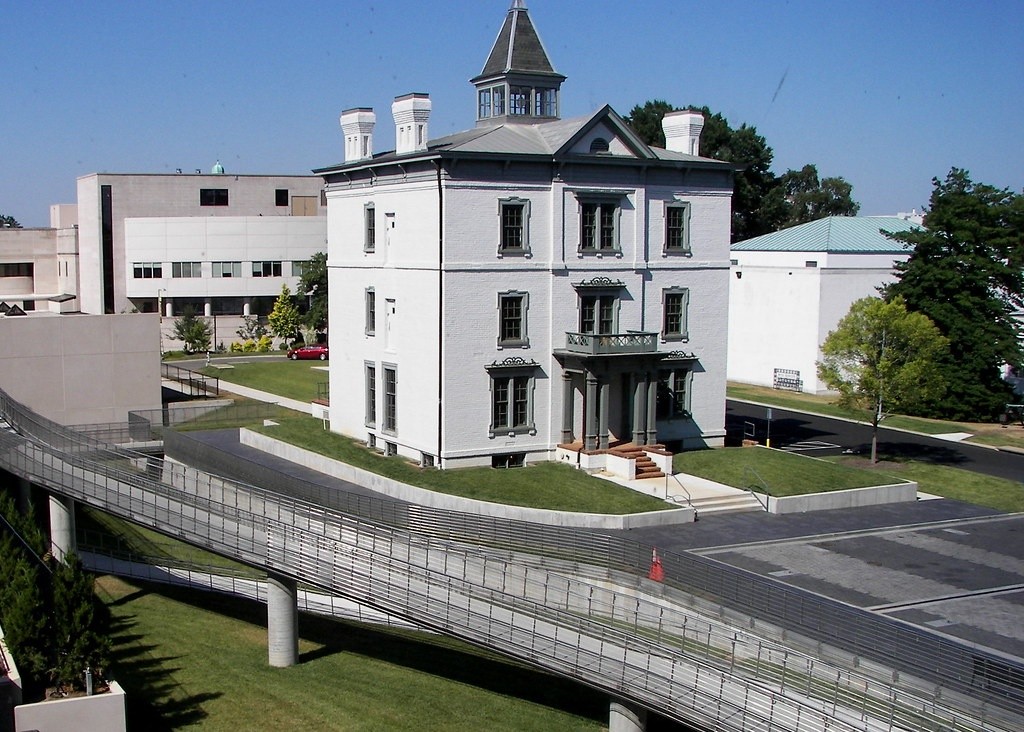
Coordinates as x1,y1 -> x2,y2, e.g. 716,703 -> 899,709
648,547 -> 665,582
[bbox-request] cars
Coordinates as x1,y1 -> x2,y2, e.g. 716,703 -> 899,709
286,344 -> 329,361
